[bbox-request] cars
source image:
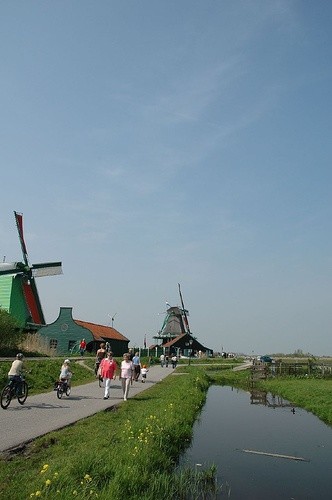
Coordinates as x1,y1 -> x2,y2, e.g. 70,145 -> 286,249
256,355 -> 273,363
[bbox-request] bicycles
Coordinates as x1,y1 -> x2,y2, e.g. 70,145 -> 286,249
53,373 -> 75,399
0,370 -> 32,409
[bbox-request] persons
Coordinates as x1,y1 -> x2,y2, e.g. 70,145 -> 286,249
221,352 -> 234,358
165,355 -> 169,368
58,359 -> 72,391
198,350 -> 202,358
8,353 -> 26,398
160,354 -> 164,367
118,353 -> 135,401
132,352 -> 141,381
79,339 -> 86,357
97,343 -> 110,362
171,354 -> 177,368
98,351 -> 117,399
141,365 -> 148,383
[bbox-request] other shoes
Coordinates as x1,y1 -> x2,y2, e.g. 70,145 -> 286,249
104,397 -> 109,399
124,398 -> 127,401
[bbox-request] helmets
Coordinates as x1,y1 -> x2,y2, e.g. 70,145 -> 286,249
64,359 -> 70,364
17,354 -> 23,359
